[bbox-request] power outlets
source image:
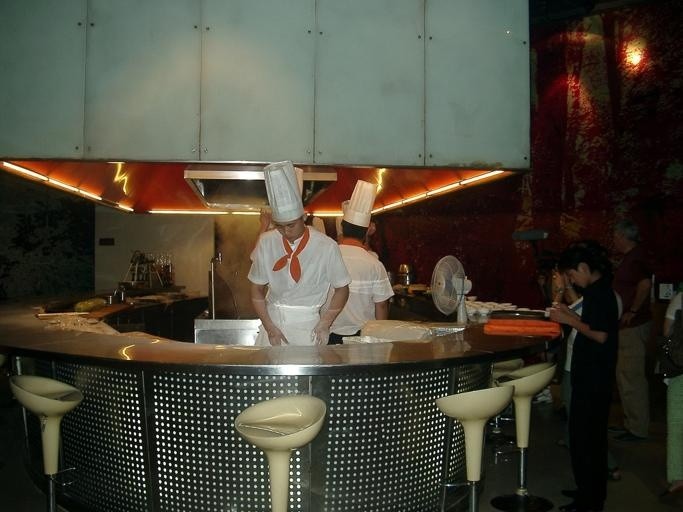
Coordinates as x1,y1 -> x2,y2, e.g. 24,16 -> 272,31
659,283 -> 673,298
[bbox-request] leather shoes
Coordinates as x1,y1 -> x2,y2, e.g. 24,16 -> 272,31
614,431 -> 648,444
561,489 -> 577,497
559,502 -> 575,512
609,424 -> 626,433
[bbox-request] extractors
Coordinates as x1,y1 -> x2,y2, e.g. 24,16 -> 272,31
183,164 -> 338,213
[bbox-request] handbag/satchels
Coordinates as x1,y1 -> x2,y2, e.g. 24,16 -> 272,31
654,305 -> 682,377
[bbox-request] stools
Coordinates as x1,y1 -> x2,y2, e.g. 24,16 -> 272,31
496,362 -> 563,512
434,384 -> 514,511
491,358 -> 524,438
232,394 -> 327,510
10,373 -> 79,512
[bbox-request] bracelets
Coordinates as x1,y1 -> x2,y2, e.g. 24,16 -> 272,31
628,308 -> 637,314
555,288 -> 565,293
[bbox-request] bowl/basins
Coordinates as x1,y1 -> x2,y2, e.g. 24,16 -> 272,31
465,296 -> 516,317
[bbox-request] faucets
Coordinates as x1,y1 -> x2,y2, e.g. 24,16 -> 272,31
211,251 -> 223,267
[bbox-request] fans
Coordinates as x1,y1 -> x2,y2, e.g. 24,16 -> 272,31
430,256 -> 473,332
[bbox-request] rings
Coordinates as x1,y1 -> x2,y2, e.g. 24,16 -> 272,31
552,275 -> 556,279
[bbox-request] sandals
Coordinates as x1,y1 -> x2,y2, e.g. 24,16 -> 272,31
607,466 -> 621,481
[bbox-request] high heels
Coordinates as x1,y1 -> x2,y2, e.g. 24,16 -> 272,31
660,486 -> 681,506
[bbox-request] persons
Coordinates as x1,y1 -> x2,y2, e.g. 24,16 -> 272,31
320,178 -> 394,345
661,290 -> 683,458
610,219 -> 653,443
552,269 -> 583,398
247,161 -> 349,346
551,243 -> 620,500
251,206 -> 326,261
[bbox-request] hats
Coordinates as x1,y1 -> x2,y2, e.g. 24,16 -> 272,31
342,178 -> 378,229
263,159 -> 303,223
